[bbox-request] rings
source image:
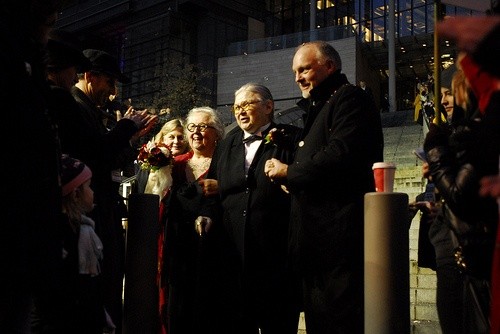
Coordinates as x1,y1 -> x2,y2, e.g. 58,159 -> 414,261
144,128 -> 146,131
197,223 -> 201,226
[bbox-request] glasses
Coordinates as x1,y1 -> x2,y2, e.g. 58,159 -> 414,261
233,99 -> 268,114
186,123 -> 216,133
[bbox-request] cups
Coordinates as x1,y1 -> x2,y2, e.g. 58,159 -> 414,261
372,162 -> 396,192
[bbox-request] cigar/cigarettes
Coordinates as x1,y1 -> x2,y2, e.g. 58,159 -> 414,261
132,109 -> 135,112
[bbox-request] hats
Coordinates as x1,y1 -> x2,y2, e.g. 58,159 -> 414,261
74,48 -> 118,77
57,153 -> 93,197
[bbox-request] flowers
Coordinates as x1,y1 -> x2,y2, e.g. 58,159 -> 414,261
137,140 -> 171,172
265,130 -> 273,144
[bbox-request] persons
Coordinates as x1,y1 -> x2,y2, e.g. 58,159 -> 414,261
162,105 -> 224,334
197,84 -> 306,334
0,0 -> 190,334
264,41 -> 383,334
410,0 -> 500,334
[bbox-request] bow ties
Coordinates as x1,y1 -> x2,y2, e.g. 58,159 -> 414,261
243,134 -> 264,143
